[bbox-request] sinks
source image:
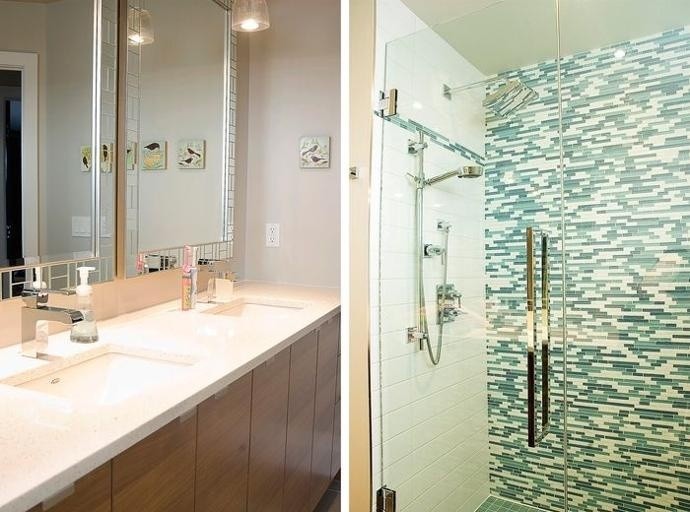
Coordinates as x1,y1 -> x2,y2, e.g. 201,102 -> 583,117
199,295 -> 313,326
0,342 -> 203,408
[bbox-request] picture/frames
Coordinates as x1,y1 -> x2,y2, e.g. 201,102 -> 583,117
101,142 -> 113,173
299,135 -> 330,169
79,145 -> 91,172
176,139 -> 205,169
126,141 -> 136,169
140,140 -> 167,170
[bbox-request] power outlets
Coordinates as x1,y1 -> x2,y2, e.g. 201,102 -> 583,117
265,223 -> 279,247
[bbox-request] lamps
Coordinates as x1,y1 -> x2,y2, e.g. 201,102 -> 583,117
128,8 -> 155,47
232,0 -> 270,32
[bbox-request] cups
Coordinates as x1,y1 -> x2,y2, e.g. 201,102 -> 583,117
216,279 -> 234,304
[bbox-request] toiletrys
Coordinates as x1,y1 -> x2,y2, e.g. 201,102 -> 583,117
32,268 -> 47,293
69,267 -> 99,344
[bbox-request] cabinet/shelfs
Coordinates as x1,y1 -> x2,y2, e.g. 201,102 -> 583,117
26,458 -> 112,512
330,314 -> 341,483
246,344 -> 291,512
309,315 -> 340,512
193,369 -> 253,510
284,328 -> 319,512
110,405 -> 198,512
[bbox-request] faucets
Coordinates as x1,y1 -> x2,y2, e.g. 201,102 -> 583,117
144,254 -> 177,273
21,286 -> 85,361
203,259 -> 230,304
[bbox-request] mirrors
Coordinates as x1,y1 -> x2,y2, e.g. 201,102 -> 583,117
124,1 -> 240,277
0,0 -> 118,301
